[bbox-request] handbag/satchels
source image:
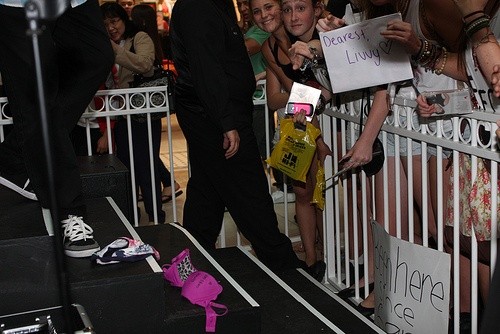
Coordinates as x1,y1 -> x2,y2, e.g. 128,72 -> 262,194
129,36 -> 177,123
265,119 -> 321,183
310,166 -> 325,211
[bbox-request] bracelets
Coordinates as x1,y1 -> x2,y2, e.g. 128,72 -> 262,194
411,37 -> 446,75
462,10 -> 499,65
300,48 -> 320,71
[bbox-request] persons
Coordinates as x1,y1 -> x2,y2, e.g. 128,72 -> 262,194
425,93 -> 449,114
169,0 -> 327,284
492,64 -> 500,138
70,0 -> 182,224
236,0 -> 431,287
316,0 -> 465,313
0,0 -> 116,257
415,0 -> 500,325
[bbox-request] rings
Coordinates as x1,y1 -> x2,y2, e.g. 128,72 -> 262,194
360,162 -> 363,164
355,162 -> 360,164
349,157 -> 354,163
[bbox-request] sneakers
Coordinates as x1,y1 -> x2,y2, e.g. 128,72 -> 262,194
62,214 -> 100,257
0,168 -> 38,201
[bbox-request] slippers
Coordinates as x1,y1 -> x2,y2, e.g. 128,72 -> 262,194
161,190 -> 184,203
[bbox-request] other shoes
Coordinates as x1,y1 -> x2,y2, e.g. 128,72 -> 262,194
311,260 -> 326,282
338,282 -> 373,297
357,303 -> 375,317
